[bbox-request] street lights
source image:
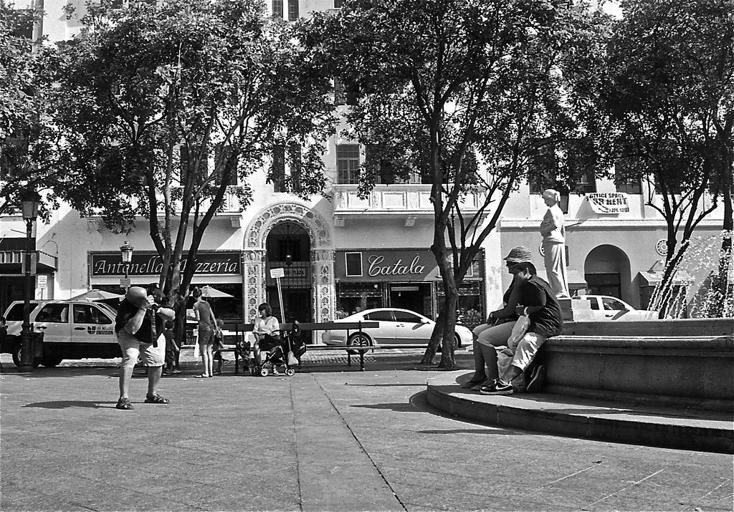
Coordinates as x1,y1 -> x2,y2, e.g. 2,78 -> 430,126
22,184 -> 40,297
120,240 -> 135,296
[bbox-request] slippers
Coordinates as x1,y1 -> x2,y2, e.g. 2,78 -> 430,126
195,373 -> 208,378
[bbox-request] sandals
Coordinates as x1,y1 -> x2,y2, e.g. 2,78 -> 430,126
116,396 -> 134,409
144,393 -> 169,403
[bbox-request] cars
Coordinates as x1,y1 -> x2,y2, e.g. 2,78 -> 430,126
572,295 -> 674,322
320,308 -> 476,352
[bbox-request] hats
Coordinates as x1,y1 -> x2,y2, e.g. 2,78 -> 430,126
503,246 -> 532,263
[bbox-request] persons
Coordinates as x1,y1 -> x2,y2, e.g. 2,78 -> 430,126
479,262 -> 563,395
253,302 -> 281,366
208,319 -> 224,372
115,286 -> 175,410
146,283 -> 186,376
540,189 -> 571,298
462,246 -> 534,391
187,287 -> 219,378
0,316 -> 8,335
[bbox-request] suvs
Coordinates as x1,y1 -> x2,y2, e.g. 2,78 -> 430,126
2,299 -> 125,366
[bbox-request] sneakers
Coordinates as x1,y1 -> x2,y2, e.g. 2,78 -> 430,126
162,371 -> 172,376
459,374 -> 487,388
480,378 -> 514,395
171,369 -> 182,374
525,360 -> 545,394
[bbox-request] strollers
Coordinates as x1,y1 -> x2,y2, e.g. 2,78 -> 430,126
247,320 -> 304,377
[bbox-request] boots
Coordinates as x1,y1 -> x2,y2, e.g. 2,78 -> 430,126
471,377 -> 499,390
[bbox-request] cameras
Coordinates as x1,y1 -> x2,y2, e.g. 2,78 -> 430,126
147,282 -> 175,310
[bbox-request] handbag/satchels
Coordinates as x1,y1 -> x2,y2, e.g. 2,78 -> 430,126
489,307 -> 518,326
508,307 -> 531,354
